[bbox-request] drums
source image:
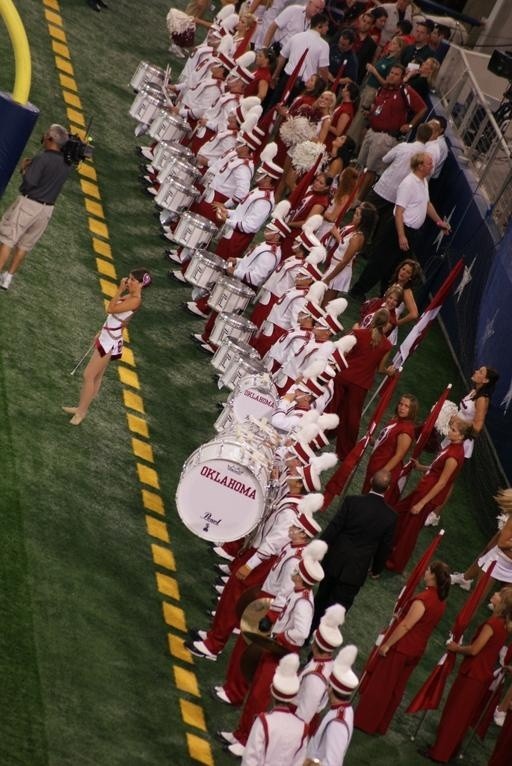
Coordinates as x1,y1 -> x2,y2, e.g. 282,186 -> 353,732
220,412 -> 236,429
147,108 -> 192,144
141,81 -> 169,101
156,153 -> 202,185
246,412 -> 281,445
207,311 -> 257,347
212,402 -> 233,433
221,352 -> 267,391
228,382 -> 278,422
129,59 -> 172,92
153,174 -> 201,214
128,90 -> 167,125
237,372 -> 279,399
210,425 -> 275,472
210,335 -> 262,373
150,138 -> 194,170
206,273 -> 257,316
172,210 -> 219,251
174,440 -> 269,545
183,248 -> 229,291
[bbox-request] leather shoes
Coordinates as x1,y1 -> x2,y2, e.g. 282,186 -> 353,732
136,129 -> 233,393
180,543 -> 247,759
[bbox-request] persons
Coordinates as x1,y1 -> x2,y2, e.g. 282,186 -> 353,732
0,121 -> 76,289
60,268 -> 152,426
136,1 -> 512,766
86,0 -> 107,12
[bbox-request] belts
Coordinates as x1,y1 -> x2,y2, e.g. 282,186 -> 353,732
23,193 -> 56,206
371,127 -> 405,142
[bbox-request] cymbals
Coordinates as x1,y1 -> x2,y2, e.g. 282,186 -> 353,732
239,596 -> 279,635
242,629 -> 289,656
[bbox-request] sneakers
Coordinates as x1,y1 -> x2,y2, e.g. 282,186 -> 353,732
1,271 -> 12,289
423,513 -> 440,527
449,573 -> 473,590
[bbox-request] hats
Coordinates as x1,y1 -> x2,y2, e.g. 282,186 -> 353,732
264,301 -> 360,706
211,4 -> 325,283
